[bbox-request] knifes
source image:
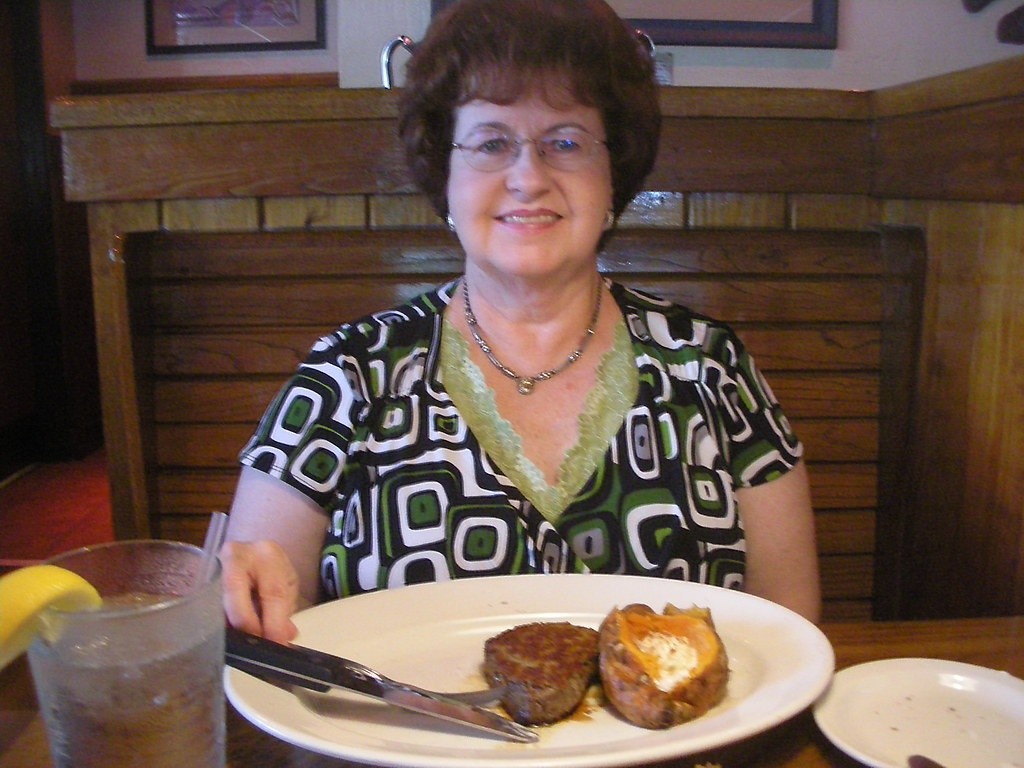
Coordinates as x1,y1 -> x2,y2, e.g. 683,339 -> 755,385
224,627 -> 540,741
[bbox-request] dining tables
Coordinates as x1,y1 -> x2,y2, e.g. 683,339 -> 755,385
1,614 -> 1024,768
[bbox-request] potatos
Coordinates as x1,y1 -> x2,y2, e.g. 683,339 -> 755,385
598,602 -> 730,730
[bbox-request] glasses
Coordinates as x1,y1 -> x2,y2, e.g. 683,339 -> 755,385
449,127 -> 611,172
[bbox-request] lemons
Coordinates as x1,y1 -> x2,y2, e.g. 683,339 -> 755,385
0,566 -> 105,668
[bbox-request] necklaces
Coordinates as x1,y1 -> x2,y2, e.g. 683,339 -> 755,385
462,277 -> 604,395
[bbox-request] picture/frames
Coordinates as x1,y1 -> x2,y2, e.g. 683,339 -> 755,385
430,0 -> 837,49
145,0 -> 326,56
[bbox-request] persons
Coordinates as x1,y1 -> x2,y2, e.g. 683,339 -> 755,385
216,0 -> 823,651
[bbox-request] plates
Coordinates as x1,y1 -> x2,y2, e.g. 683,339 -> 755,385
810,657 -> 1024,768
223,574 -> 836,768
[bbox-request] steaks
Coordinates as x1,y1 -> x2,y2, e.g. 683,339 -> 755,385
483,623 -> 600,723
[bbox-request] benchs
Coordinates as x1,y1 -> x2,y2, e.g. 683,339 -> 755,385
124,225 -> 926,623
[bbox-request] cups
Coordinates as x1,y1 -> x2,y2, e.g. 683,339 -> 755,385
25,539 -> 227,768
